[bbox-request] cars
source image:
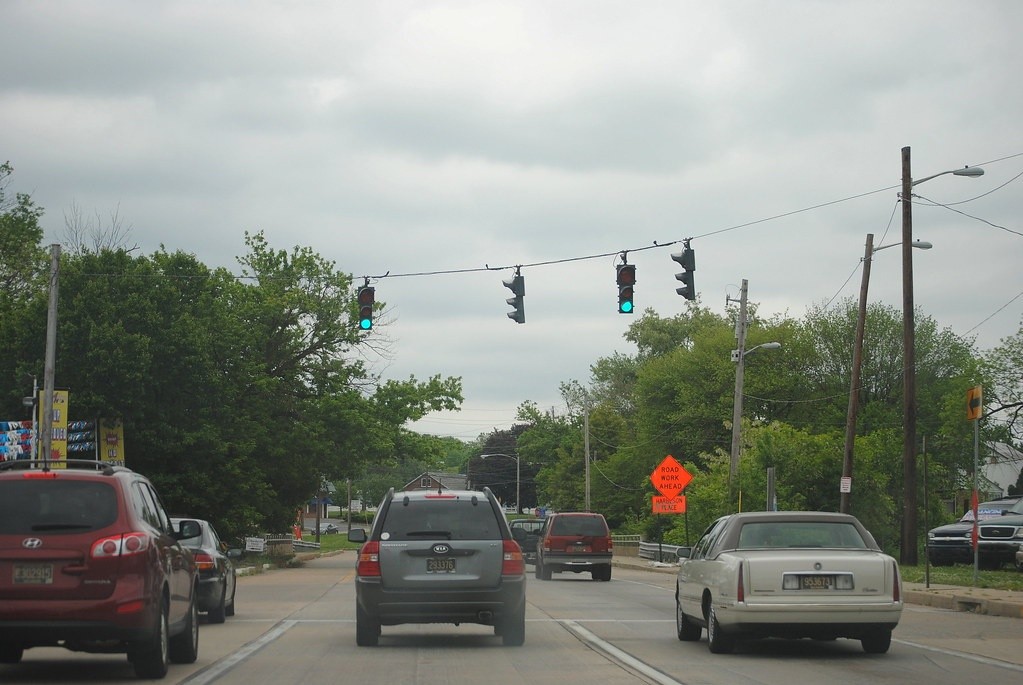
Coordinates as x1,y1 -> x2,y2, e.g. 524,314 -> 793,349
673,511 -> 904,653
169,518 -> 242,624
310,523 -> 340,535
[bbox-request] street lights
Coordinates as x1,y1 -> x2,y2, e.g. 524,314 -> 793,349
21,375 -> 39,494
425,459 -> 445,491
727,341 -> 784,517
479,451 -> 521,517
899,147 -> 985,567
840,233 -> 933,513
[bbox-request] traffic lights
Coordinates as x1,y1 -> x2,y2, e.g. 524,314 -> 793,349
357,286 -> 375,331
616,264 -> 636,314
502,276 -> 525,324
670,249 -> 696,301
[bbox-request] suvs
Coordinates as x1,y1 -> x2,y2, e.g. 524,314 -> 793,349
923,494 -> 1022,567
347,487 -> 528,646
979,496 -> 1023,571
533,510 -> 613,582
508,518 -> 548,563
0,458 -> 204,680
